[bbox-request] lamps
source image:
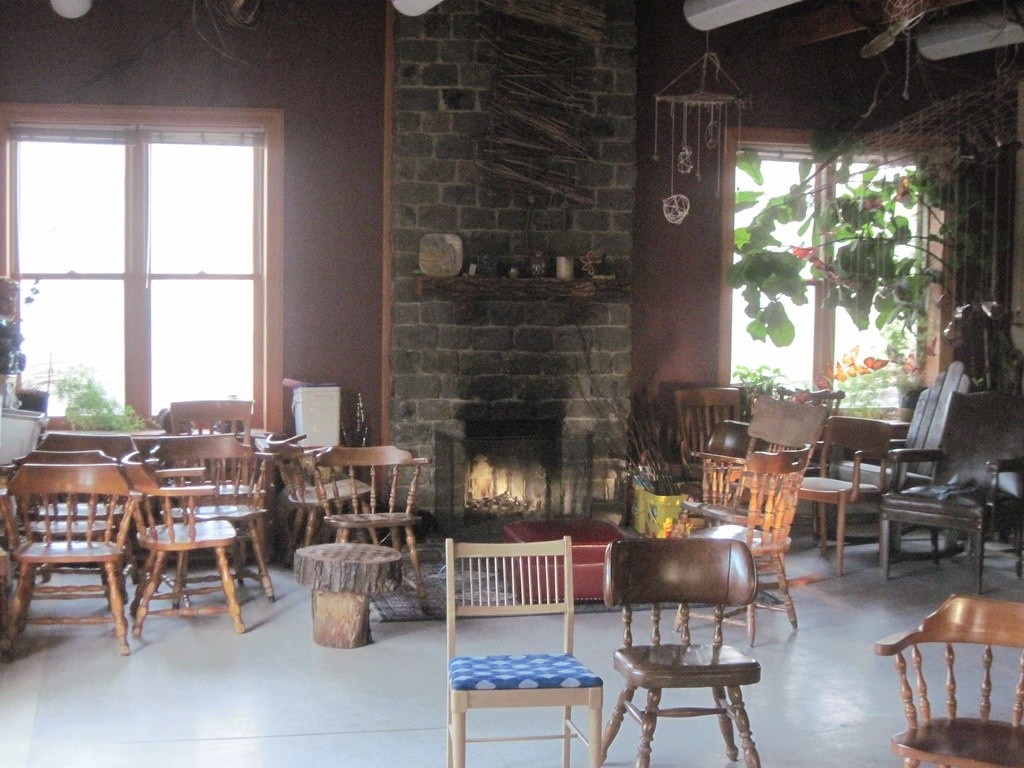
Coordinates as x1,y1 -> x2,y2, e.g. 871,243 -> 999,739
915,4 -> 1024,60
685,0 -> 803,30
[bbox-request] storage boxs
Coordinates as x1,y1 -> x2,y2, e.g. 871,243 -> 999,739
0,407 -> 50,466
294,387 -> 340,446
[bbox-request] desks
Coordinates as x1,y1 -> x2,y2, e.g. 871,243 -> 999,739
43,430 -> 267,442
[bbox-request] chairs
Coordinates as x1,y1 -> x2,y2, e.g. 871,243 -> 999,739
881,391 -> 1024,594
875,596 -> 1024,768
266,435 -> 379,565
675,387 -> 848,553
675,443 -> 810,646
813,361 -> 971,563
600,538 -> 764,767
310,445 -> 435,613
785,415 -> 893,577
0,400 -> 278,659
445,536 -> 605,767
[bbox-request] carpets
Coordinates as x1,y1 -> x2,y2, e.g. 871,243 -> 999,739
372,541 -> 785,625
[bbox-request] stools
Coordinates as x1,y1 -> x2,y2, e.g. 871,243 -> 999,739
503,520 -> 629,602
294,544 -> 404,649
